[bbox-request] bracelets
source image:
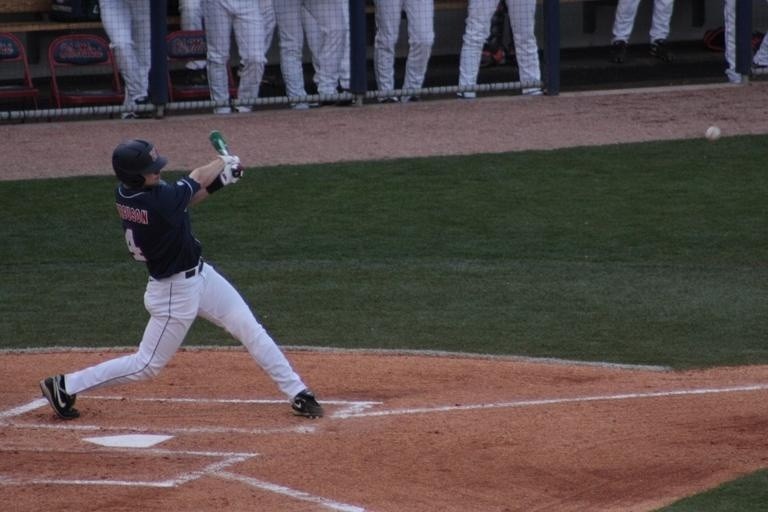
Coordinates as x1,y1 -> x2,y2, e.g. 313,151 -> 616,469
205,175 -> 222,193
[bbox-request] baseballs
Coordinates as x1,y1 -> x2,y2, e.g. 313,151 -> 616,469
705,126 -> 721,142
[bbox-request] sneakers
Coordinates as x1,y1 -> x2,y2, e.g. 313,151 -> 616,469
38,374 -> 81,420
606,41 -> 629,64
291,388 -> 324,420
648,40 -> 680,65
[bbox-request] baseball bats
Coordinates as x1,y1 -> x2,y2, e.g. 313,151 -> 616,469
208,130 -> 240,178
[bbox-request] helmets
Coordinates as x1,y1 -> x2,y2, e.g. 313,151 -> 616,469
110,138 -> 168,182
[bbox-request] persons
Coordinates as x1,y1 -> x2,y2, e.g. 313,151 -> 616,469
611,1 -> 675,68
39,140 -> 324,419
99,0 -> 566,122
751,23 -> 767,74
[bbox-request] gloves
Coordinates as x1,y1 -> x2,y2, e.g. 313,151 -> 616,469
217,153 -> 242,166
219,162 -> 245,187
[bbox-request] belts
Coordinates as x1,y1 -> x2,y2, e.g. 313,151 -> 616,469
185,260 -> 203,280
